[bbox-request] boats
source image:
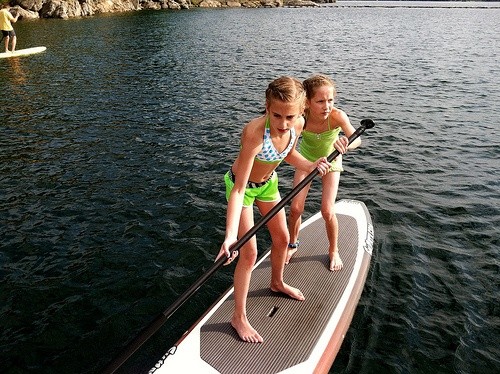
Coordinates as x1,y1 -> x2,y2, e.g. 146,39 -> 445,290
0,46 -> 47,58
143,198 -> 374,374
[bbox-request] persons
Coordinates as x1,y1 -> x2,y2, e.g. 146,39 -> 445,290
281,74 -> 362,272
0,3 -> 20,52
213,73 -> 332,343
